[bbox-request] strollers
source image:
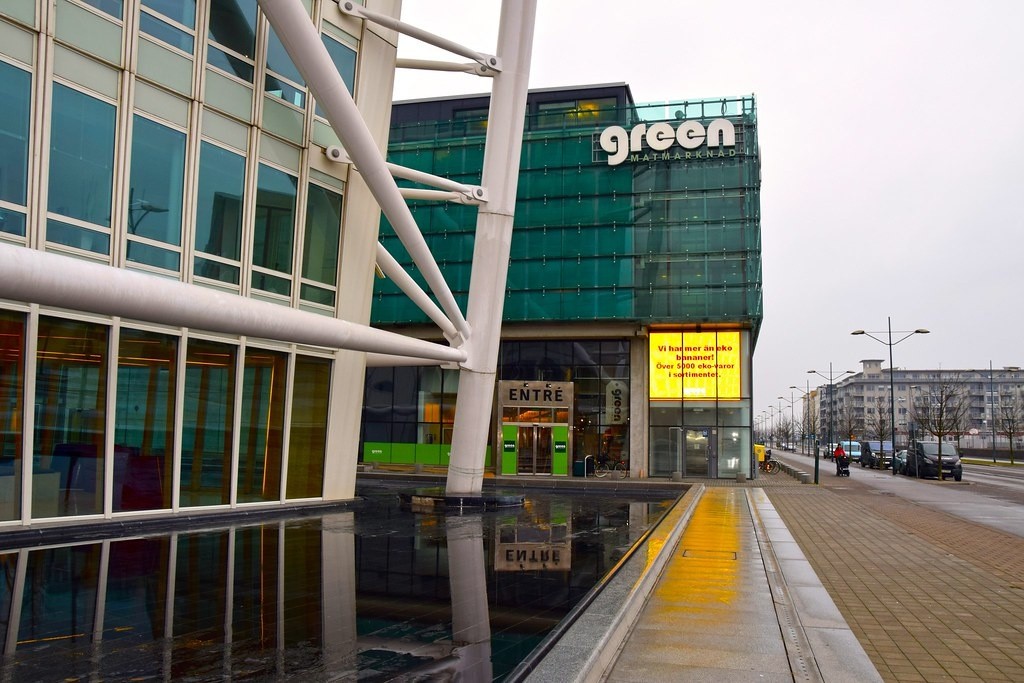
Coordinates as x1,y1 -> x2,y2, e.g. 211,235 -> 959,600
837,455 -> 850,477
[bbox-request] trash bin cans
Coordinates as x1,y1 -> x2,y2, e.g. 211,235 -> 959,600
574,456 -> 595,477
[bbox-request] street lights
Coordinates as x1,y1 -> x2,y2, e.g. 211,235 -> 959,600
850,316 -> 931,476
763,408 -> 781,449
768,400 -> 791,449
778,391 -> 808,453
964,359 -> 1014,463
790,379 -> 827,457
910,381 -> 947,440
807,362 -> 854,462
754,410 -> 780,449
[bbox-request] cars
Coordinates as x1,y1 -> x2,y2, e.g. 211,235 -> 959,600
824,442 -> 839,459
780,441 -> 798,452
894,449 -> 908,476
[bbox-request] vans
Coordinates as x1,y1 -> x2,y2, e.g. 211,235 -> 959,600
906,439 -> 963,480
840,440 -> 862,463
859,441 -> 893,470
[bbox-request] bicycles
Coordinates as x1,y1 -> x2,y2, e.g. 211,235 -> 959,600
594,458 -> 628,479
758,450 -> 782,475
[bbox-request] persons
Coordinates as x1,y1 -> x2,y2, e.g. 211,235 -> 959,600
834,443 -> 845,476
